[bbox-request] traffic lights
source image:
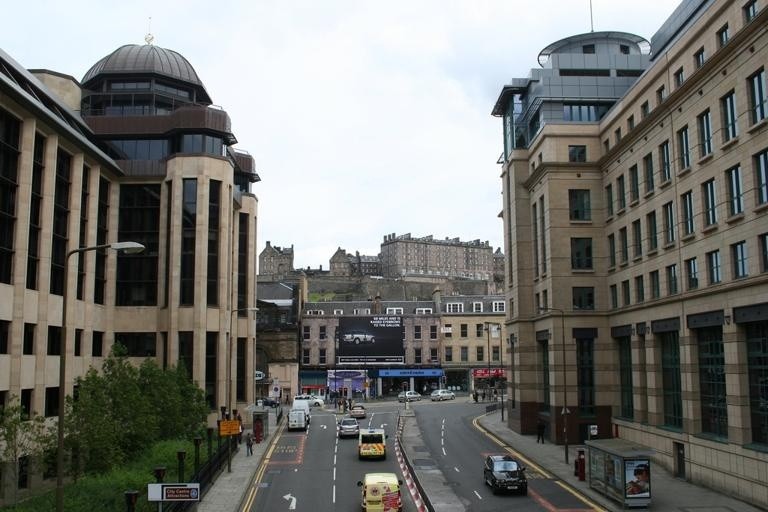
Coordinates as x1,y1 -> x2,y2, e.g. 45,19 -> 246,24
442,376 -> 446,384
403,383 -> 407,391
325,387 -> 328,394
344,388 -> 347,396
339,388 -> 342,396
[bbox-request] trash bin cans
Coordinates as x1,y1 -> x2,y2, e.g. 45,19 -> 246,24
574,460 -> 580,477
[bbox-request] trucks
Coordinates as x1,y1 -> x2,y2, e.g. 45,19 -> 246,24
358,426 -> 389,461
357,472 -> 403,511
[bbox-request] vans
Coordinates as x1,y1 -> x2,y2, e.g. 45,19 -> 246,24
286,393 -> 324,432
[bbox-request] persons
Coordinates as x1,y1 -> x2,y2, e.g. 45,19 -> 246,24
535,418 -> 545,444
237,413 -> 244,444
286,394 -> 290,405
330,390 -> 352,410
246,433 -> 254,458
473,389 -> 498,402
626,464 -> 649,495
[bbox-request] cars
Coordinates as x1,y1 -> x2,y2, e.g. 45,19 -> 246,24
256,396 -> 280,408
430,389 -> 455,401
350,405 -> 367,418
398,390 -> 422,402
343,330 -> 376,345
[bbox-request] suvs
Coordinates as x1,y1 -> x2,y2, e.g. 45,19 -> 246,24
337,417 -> 360,438
482,454 -> 529,498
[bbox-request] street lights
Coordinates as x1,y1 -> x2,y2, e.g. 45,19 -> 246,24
56,241 -> 148,511
536,306 -> 570,464
484,320 -> 504,421
226,306 -> 260,475
